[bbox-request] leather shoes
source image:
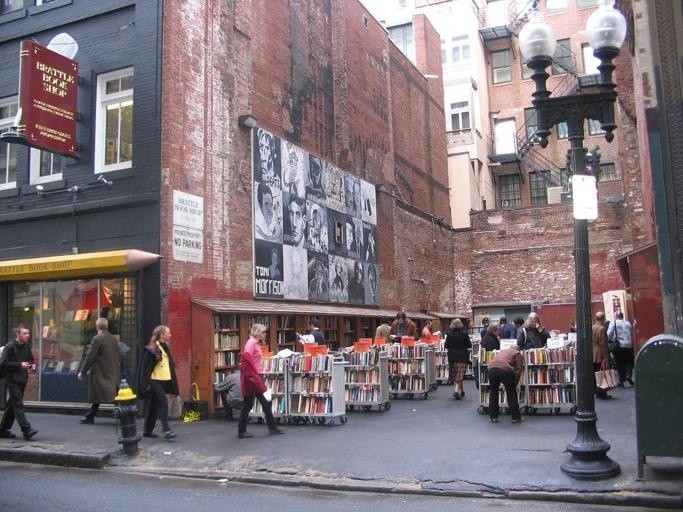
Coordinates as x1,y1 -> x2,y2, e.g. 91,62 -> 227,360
165,431 -> 177,438
78,417 -> 93,424
23,429 -> 39,439
143,432 -> 158,438
1,431 -> 15,439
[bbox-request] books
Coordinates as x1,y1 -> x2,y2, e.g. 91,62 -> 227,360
302,316 -> 317,335
248,315 -> 269,345
214,314 -> 243,407
248,353 -> 336,412
277,314 -> 297,351
433,340 -> 449,378
324,317 -> 370,350
480,347 -> 576,404
338,345 -> 385,401
387,342 -> 429,391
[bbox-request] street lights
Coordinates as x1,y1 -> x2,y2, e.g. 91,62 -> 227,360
518,1 -> 627,481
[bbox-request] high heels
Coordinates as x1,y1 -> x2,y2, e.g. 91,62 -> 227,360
461,392 -> 465,397
453,392 -> 461,401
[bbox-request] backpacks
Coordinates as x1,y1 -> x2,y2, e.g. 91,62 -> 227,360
0,340 -> 21,379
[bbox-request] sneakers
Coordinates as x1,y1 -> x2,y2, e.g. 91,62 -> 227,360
596,375 -> 634,399
269,427 -> 286,434
512,417 -> 524,423
238,432 -> 254,438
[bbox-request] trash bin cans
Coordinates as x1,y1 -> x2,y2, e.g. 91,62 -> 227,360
634,333 -> 683,482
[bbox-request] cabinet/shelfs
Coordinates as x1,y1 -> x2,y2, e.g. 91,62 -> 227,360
428,312 -> 469,338
192,307 -> 424,418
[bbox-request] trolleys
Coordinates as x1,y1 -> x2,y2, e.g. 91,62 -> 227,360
246,352 -> 349,427
427,333 -> 484,387
373,342 -> 433,400
327,346 -> 392,413
477,340 -> 577,418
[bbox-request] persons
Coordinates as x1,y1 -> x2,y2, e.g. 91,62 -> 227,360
375,316 -> 392,343
481,316 -> 524,351
136,325 -> 179,439
307,318 -> 325,345
518,312 -> 551,349
253,126 -> 379,306
445,318 -> 472,399
77,318 -> 121,424
422,320 -> 432,336
214,364 -> 245,413
592,311 -> 634,400
391,311 -> 416,342
483,346 -> 526,423
238,324 -> 284,439
0,326 -> 38,440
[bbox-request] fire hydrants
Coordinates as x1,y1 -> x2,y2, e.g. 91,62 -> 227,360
113,379 -> 143,456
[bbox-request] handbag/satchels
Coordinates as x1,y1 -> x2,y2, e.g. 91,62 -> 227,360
520,328 -> 532,349
594,359 -> 619,390
607,320 -> 621,352
